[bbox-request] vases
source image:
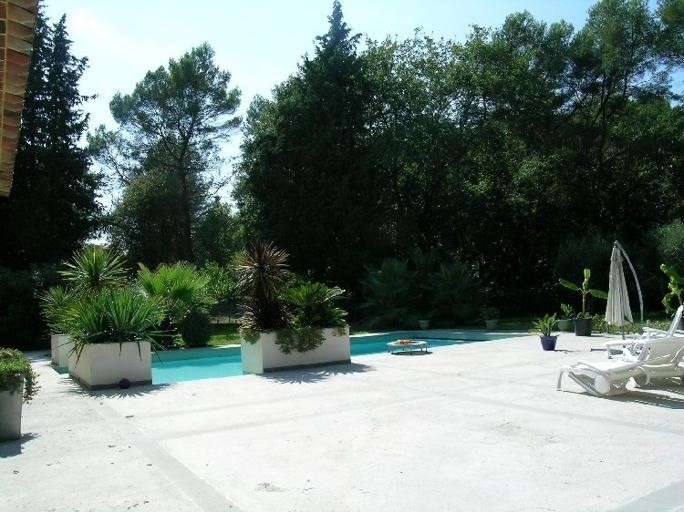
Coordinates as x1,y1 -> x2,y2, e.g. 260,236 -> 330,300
418,320 -> 429,329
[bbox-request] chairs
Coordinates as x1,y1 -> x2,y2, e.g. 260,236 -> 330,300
555,303 -> 683,398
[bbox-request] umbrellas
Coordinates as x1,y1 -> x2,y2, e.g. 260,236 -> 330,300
604,242 -> 645,330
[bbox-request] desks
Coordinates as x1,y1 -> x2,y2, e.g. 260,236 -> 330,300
388,339 -> 427,354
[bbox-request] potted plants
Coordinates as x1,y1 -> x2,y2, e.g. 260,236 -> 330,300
532,311 -> 560,350
237,281 -> 351,372
479,307 -> 499,331
0,349 -> 36,442
36,284 -> 166,391
